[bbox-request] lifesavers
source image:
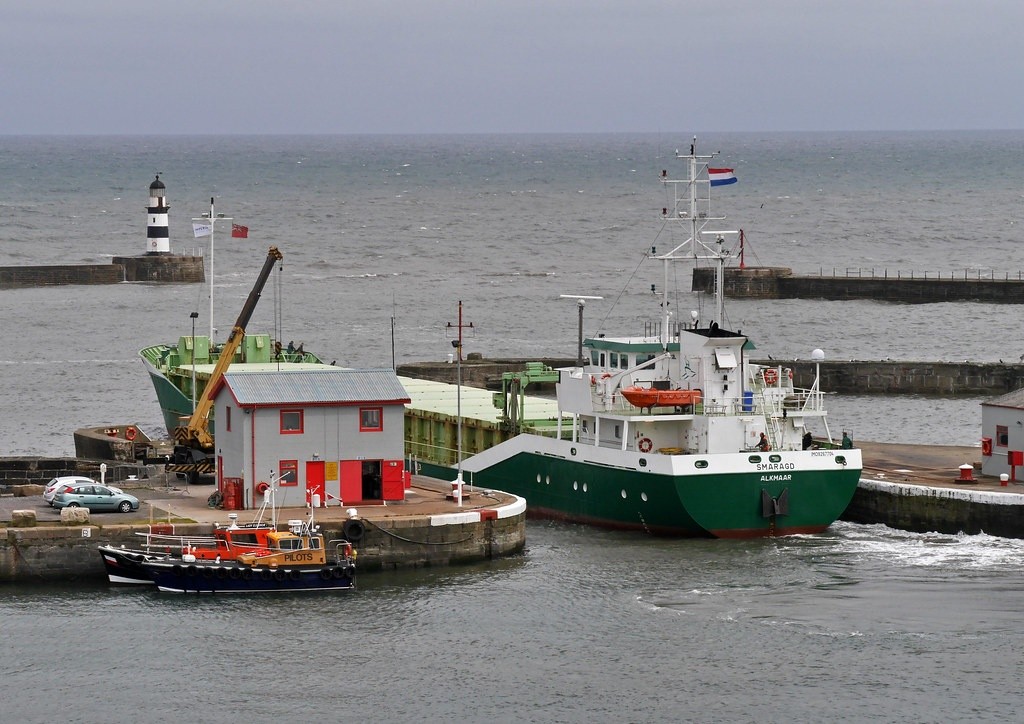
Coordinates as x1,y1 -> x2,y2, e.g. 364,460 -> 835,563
257,482 -> 270,495
639,436 -> 652,452
765,369 -> 778,384
343,543 -> 352,557
152,241 -> 157,247
126,426 -> 138,441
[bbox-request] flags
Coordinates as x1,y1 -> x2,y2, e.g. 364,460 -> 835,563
192,223 -> 212,238
232,224 -> 248,238
707,167 -> 737,187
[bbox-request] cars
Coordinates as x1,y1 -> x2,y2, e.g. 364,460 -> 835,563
51,483 -> 140,513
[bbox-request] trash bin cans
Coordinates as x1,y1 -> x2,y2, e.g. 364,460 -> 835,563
743,391 -> 754,411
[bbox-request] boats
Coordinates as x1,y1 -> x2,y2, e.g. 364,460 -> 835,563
139,136 -> 863,540
619,386 -> 702,408
97,468 -> 358,593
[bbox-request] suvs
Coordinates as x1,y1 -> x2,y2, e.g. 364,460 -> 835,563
42,475 -> 124,507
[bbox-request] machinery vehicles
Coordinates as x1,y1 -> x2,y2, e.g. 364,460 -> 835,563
162,245 -> 286,485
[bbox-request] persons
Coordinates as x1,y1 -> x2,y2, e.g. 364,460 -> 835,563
755,433 -> 769,452
803,431 -> 812,450
288,340 -> 304,361
842,432 -> 852,449
